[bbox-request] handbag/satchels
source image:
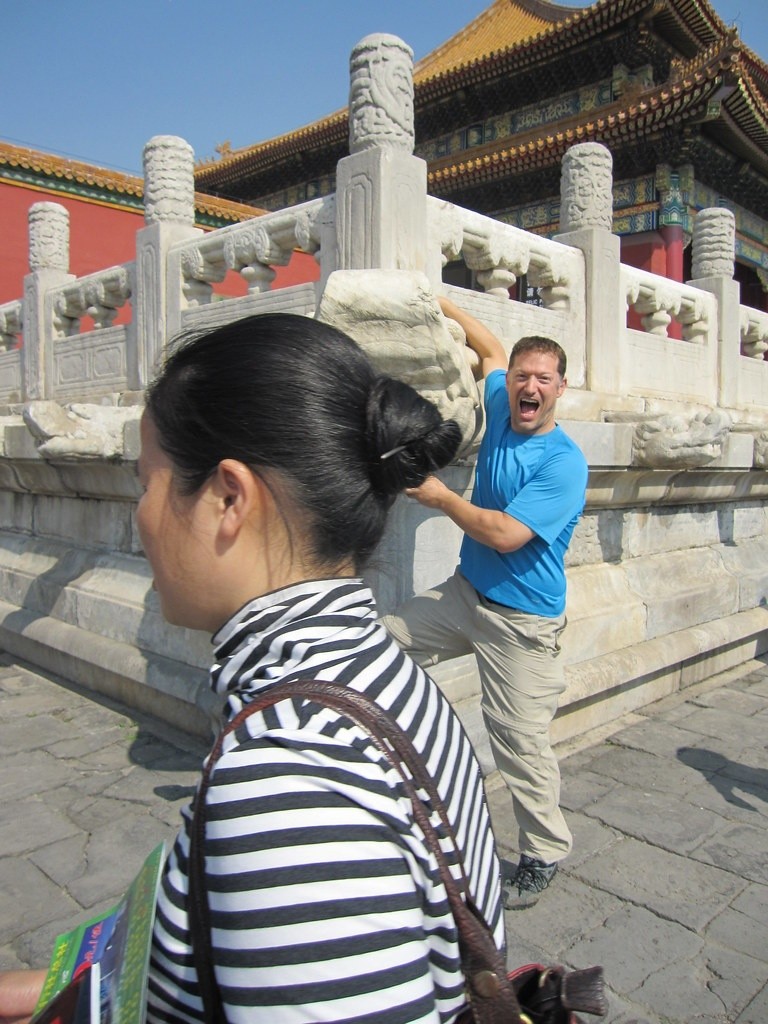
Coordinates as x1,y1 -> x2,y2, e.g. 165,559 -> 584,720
458,963 -> 605,1024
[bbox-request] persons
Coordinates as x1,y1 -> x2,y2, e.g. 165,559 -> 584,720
377,299 -> 593,914
1,312 -> 506,1024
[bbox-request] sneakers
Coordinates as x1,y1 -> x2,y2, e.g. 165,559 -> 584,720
501,855 -> 558,911
